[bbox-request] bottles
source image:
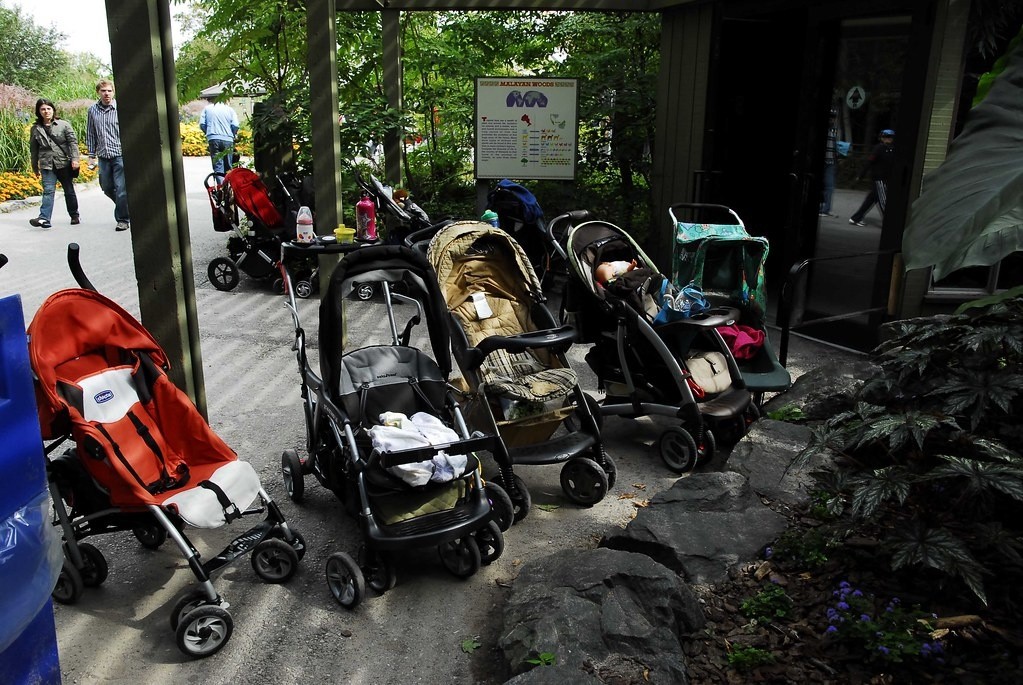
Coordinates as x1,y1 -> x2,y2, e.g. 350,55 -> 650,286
295,206 -> 314,242
481,210 -> 499,228
333,223 -> 356,244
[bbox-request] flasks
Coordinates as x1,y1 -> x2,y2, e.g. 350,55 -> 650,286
355,189 -> 375,240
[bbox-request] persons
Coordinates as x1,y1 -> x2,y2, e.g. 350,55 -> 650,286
596,259 -> 658,321
86,80 -> 131,231
393,189 -> 432,223
29,98 -> 81,228
848,129 -> 894,227
817,108 -> 842,218
200,95 -> 240,186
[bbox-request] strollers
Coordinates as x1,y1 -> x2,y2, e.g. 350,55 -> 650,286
218,141 -> 241,177
0,164 -> 792,657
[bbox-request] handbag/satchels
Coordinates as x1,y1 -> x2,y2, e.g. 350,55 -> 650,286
68,159 -> 80,178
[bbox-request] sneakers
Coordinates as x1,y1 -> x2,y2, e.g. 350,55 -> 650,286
29,218 -> 52,229
116,220 -> 130,231
69,216 -> 80,225
